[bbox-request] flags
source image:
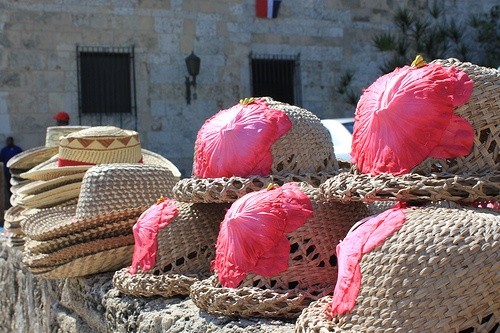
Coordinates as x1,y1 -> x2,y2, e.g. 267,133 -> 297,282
256,0 -> 281,19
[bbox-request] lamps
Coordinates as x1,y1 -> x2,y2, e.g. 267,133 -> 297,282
185,49 -> 201,104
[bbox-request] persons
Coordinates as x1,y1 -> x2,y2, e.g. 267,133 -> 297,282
0,136 -> 24,210
53,112 -> 70,126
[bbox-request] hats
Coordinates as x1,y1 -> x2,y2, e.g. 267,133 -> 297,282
113,198 -> 229,296
295,202 -> 500,333
52,112 -> 70,121
189,183 -> 374,317
4,125 -> 181,280
317,55 -> 500,203
171,97 -> 349,204
